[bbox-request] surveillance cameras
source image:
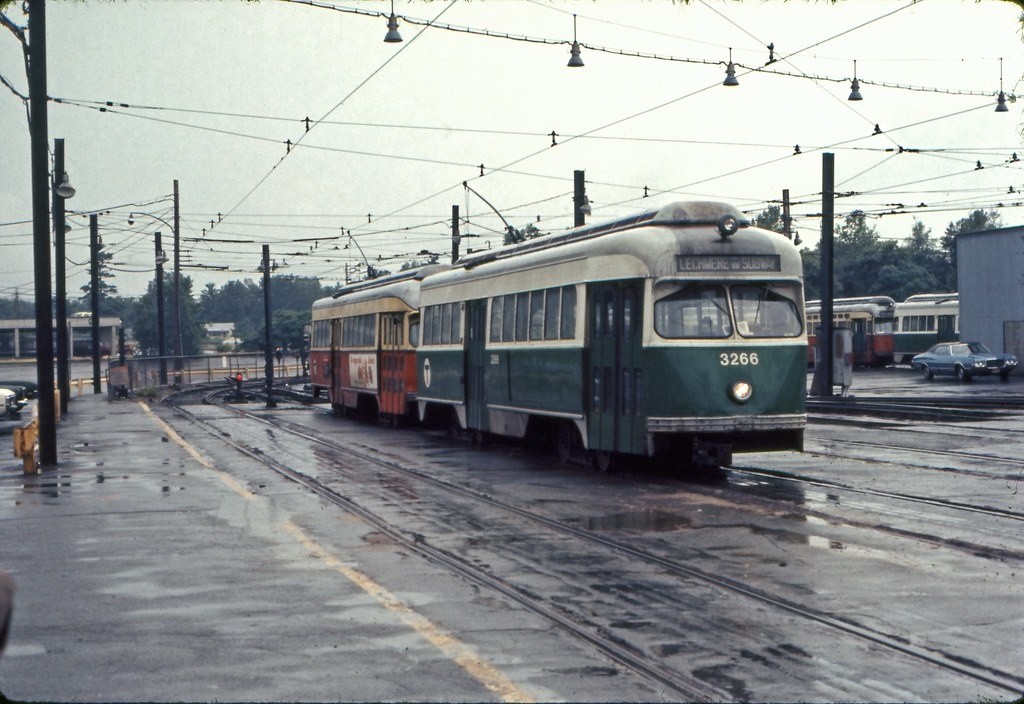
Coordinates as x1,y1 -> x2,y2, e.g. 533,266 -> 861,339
128,219 -> 134,225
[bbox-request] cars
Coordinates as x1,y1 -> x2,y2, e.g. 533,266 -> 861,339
0,388 -> 16,416
910,341 -> 1018,384
531,308 -> 542,326
0,385 -> 27,411
596,307 -> 630,327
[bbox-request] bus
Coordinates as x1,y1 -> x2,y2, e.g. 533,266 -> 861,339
413,200 -> 808,471
786,296 -> 895,369
893,293 -> 960,350
681,305 -> 760,329
308,264 -> 452,428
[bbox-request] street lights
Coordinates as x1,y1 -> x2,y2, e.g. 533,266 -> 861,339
782,190 -> 803,248
152,231 -> 169,386
50,137 -> 75,420
572,169 -> 592,227
127,181 -> 183,387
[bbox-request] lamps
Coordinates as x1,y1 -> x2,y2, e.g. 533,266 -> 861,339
994,58 -> 1009,112
384,0 -> 403,43
568,14 -> 584,66
848,60 -> 863,100
723,47 -> 739,86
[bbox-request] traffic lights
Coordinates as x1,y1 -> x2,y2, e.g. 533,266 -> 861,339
235,373 -> 243,382
